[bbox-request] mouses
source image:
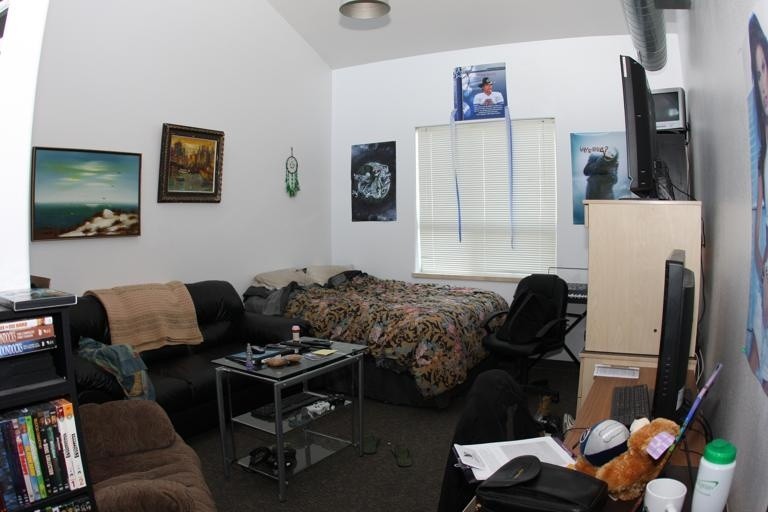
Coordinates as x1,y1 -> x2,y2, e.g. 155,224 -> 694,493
251,345 -> 265,354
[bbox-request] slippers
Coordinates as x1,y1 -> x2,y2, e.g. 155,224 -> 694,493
362,429 -> 385,454
386,435 -> 413,468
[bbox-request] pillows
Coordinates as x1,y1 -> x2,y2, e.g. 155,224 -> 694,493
248,267 -> 315,291
305,262 -> 355,288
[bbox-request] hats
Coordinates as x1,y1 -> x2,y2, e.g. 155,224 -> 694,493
477,77 -> 494,88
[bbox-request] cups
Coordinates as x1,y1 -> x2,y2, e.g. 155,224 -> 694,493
642,477 -> 687,512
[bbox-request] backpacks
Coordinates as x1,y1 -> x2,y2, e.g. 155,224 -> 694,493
472,454 -> 610,512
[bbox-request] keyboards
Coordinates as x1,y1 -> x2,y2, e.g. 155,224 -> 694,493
251,392 -> 322,422
611,384 -> 650,429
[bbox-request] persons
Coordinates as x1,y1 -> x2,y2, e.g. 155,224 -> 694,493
473,77 -> 504,106
747,15 -> 768,400
583,147 -> 618,200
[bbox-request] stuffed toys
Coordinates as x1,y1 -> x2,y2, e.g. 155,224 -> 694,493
566,417 -> 679,505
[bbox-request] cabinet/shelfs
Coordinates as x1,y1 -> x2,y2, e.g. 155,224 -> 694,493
0,300 -> 102,512
578,194 -> 704,359
570,347 -> 703,420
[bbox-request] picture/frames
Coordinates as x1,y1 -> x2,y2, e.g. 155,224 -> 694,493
30,144 -> 144,243
156,120 -> 227,206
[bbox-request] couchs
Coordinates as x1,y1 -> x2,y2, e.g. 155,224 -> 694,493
61,271 -> 315,438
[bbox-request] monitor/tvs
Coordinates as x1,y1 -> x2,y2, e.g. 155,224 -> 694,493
652,248 -> 696,423
618,55 -> 656,200
651,87 -> 687,134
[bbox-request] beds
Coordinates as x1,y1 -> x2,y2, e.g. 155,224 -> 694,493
240,260 -> 514,415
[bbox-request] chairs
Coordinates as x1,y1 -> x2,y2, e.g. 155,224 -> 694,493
435,369 -> 544,512
74,393 -> 218,511
473,270 -> 573,403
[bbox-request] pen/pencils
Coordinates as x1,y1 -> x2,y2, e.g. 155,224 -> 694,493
597,364 -> 641,371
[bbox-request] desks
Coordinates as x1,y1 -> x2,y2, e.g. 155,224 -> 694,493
560,363 -> 728,511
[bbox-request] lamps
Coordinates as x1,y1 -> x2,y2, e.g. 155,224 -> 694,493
335,0 -> 394,25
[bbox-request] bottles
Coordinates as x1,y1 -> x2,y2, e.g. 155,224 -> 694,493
245,343 -> 254,369
690,439 -> 738,512
291,325 -> 300,341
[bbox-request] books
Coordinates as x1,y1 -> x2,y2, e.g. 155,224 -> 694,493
0,398 -> 93,512
593,364 -> 640,379
